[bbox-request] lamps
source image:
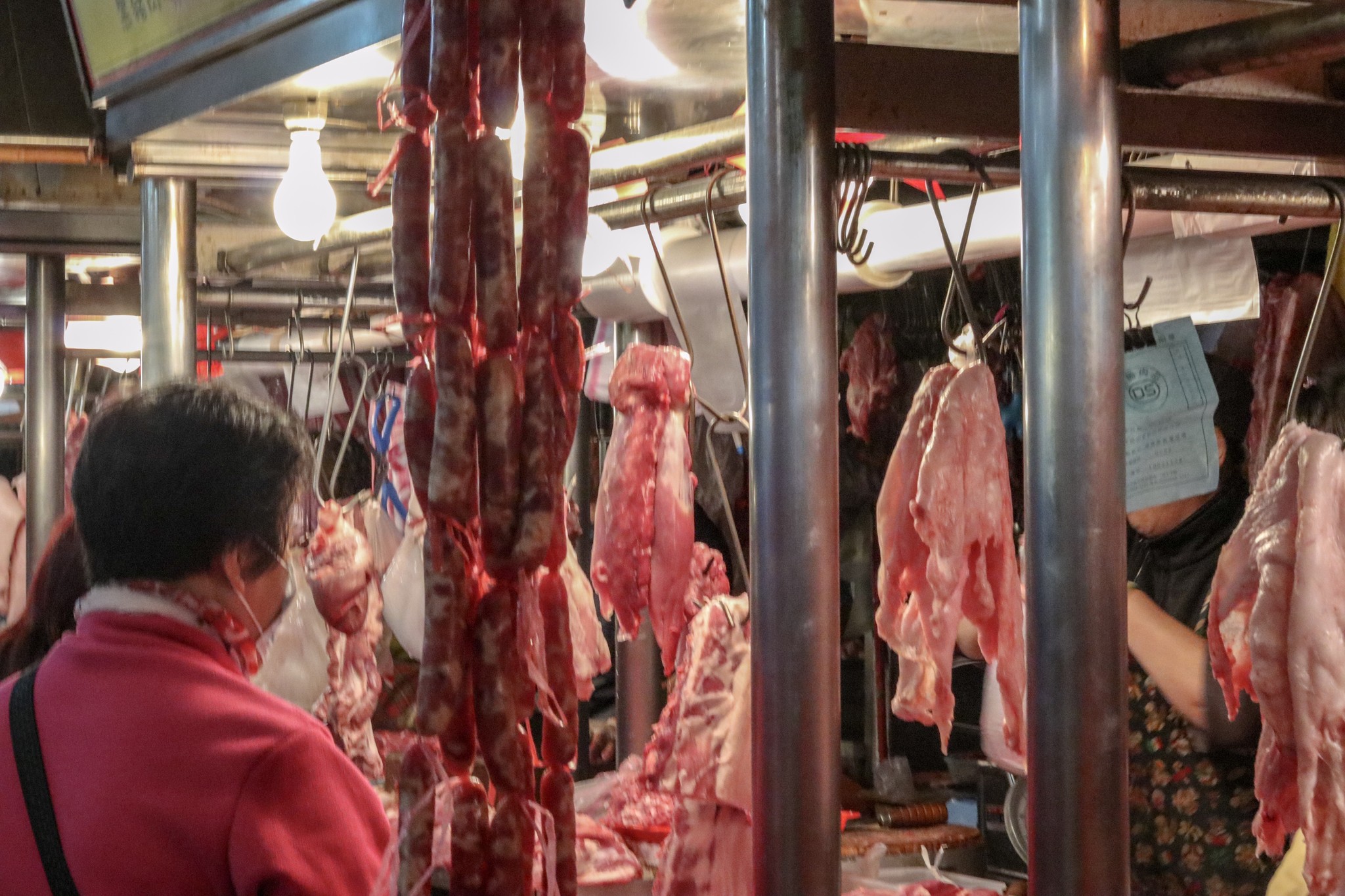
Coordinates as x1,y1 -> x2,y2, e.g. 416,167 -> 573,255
270,96 -> 340,245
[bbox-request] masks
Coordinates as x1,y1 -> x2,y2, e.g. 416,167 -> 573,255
220,531 -> 297,667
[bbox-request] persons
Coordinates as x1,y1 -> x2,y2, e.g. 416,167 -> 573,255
6,375 -> 405,895
0,517 -> 90,680
1123,415 -> 1299,895
